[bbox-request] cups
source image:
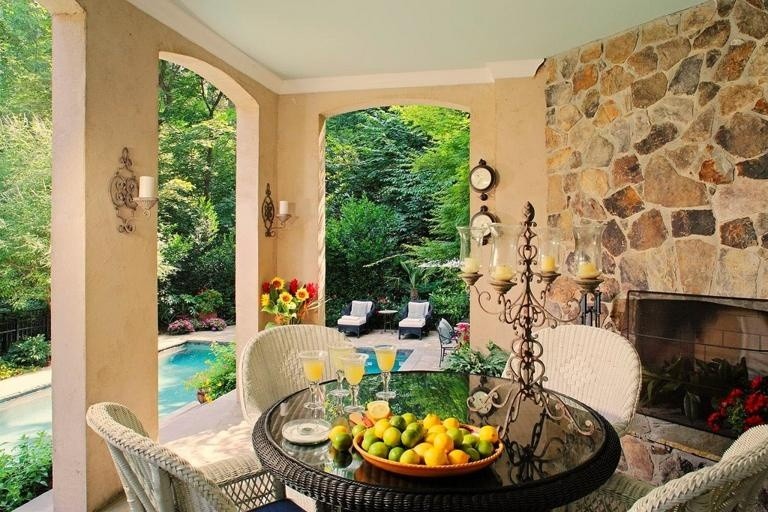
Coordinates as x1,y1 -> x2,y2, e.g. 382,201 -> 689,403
532,226 -> 565,272
455,225 -> 483,274
485,222 -> 525,283
567,224 -> 604,278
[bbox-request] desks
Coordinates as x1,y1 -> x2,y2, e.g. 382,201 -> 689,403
377,310 -> 397,335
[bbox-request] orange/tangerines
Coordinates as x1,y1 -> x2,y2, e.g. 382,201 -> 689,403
328,400 -> 499,466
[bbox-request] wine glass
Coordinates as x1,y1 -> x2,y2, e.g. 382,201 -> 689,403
373,343 -> 398,399
339,352 -> 367,412
297,349 -> 329,410
325,340 -> 355,398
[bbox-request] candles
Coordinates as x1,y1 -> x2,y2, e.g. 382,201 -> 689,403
495,264 -> 513,279
137,175 -> 155,198
463,256 -> 478,273
541,254 -> 555,272
278,200 -> 289,215
580,261 -> 597,278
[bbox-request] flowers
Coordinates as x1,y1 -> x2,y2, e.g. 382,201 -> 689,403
259,276 -> 317,328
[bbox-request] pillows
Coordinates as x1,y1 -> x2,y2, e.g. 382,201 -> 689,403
407,302 -> 425,319
349,301 -> 367,317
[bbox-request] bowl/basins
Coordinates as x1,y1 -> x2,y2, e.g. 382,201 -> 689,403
281,418 -> 333,446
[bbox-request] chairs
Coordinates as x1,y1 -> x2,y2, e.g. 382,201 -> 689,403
397,300 -> 433,340
546,421 -> 767,512
85,400 -> 306,511
335,299 -> 375,339
499,323 -> 642,437
435,318 -> 461,367
232,321 -> 354,431
